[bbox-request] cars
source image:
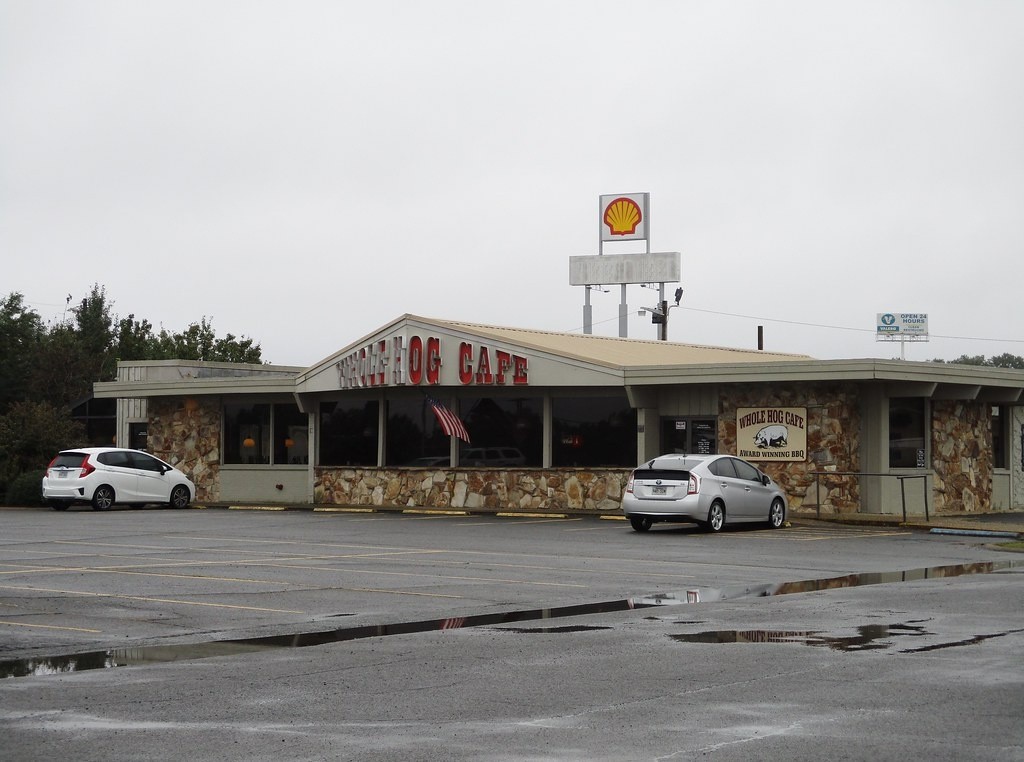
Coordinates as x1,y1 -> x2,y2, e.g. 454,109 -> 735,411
40,448 -> 196,513
622,452 -> 789,534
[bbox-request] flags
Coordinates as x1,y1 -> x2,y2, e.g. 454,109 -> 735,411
417,387 -> 470,444
